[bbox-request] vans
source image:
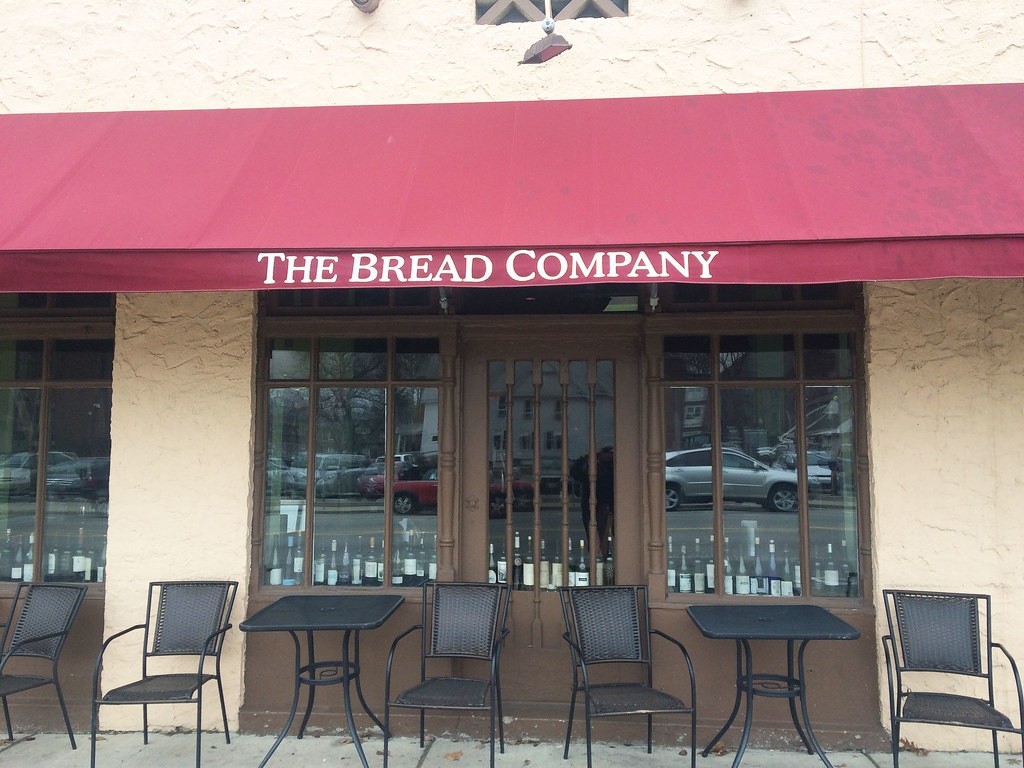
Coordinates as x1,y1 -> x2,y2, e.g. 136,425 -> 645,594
375,454 -> 415,464
288,452 -> 368,498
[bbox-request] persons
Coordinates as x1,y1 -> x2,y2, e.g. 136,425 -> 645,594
575,446 -> 616,560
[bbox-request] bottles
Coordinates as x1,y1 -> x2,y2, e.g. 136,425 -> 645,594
0,516 -> 858,600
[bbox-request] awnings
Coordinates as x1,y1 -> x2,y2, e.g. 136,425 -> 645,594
0,82 -> 1023,294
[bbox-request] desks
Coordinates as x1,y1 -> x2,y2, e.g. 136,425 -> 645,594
239,595 -> 406,768
686,605 -> 861,768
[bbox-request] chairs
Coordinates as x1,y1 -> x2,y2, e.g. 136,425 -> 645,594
384,582 -> 511,768
91,581 -> 238,768
0,582 -> 88,750
882,590 -> 1024,768
559,585 -> 696,768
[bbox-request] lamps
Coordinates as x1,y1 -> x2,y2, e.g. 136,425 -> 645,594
516,34 -> 573,67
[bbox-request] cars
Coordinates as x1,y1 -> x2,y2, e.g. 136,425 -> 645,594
664,445 -> 800,513
355,461 -> 408,498
771,451 -> 833,492
393,454 -> 533,518
47,457 -> 110,496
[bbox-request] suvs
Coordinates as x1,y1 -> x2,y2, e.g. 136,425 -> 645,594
0,449 -> 78,502
267,456 -> 295,495
